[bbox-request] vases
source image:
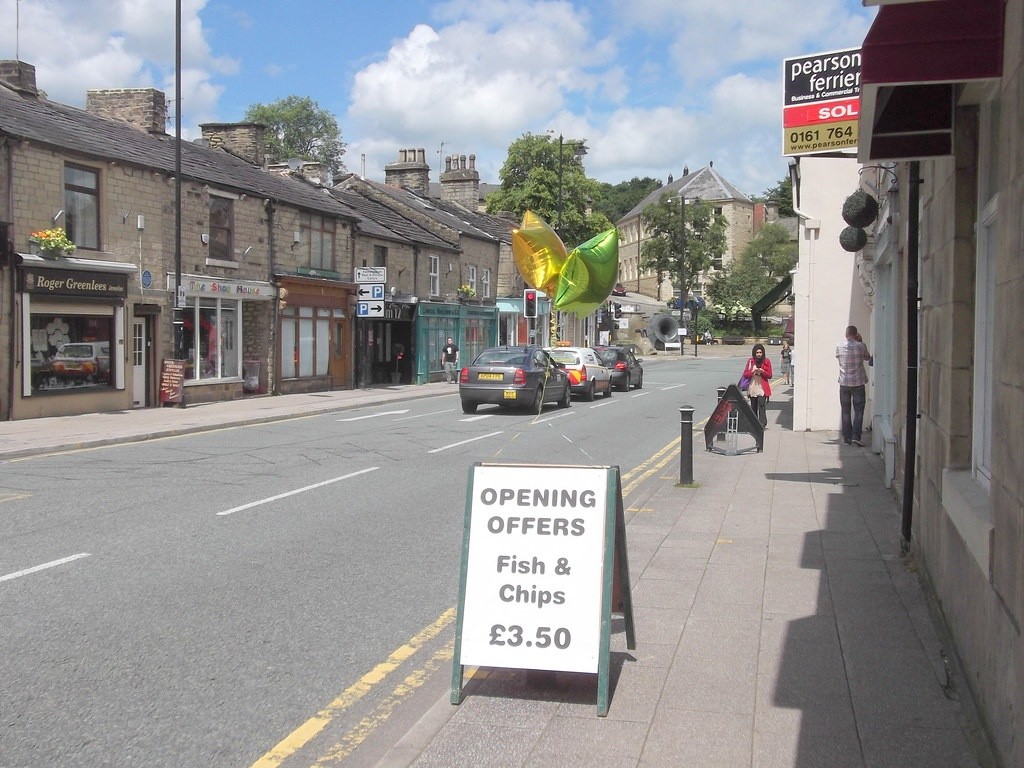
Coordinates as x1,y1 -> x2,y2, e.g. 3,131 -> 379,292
29,239 -> 58,259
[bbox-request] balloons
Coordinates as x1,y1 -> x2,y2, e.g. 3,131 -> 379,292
511,211 -> 618,320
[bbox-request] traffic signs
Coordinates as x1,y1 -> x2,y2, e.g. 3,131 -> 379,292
355,301 -> 386,318
358,283 -> 385,302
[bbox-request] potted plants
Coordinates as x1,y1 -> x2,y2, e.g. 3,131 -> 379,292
458,284 -> 475,300
389,344 -> 404,383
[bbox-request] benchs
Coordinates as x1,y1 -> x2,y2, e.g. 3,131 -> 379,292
722,336 -> 745,345
691,337 -> 718,345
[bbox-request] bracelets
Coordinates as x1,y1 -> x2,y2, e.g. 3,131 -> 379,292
455,359 -> 458,360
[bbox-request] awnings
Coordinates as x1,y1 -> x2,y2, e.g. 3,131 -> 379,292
857,0 -> 1010,164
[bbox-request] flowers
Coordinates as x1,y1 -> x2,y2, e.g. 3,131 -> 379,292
31,226 -> 76,254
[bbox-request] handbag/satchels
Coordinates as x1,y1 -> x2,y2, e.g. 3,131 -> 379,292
737,359 -> 752,391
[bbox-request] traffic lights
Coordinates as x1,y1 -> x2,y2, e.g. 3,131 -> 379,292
523,289 -> 537,319
689,299 -> 698,317
613,302 -> 622,321
614,323 -> 620,330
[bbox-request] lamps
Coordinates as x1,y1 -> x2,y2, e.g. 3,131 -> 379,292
291,232 -> 299,251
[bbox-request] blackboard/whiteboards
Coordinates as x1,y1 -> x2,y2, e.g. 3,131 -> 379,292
459,464 -> 608,672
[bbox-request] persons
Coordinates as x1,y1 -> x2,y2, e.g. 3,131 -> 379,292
779,344 -> 794,387
704,329 -> 712,345
835,325 -> 871,447
744,344 -> 773,427
441,337 -> 459,384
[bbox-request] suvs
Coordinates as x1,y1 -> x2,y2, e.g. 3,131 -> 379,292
53,341 -> 110,386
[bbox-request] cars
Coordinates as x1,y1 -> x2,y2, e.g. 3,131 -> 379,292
542,346 -> 614,403
593,347 -> 644,393
459,345 -> 572,415
612,283 -> 627,296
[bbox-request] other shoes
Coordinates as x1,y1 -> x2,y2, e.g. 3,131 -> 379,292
844,442 -> 851,446
852,439 -> 864,446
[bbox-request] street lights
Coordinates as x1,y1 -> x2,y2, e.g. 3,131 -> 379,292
667,195 -> 700,356
557,133 -> 589,240
